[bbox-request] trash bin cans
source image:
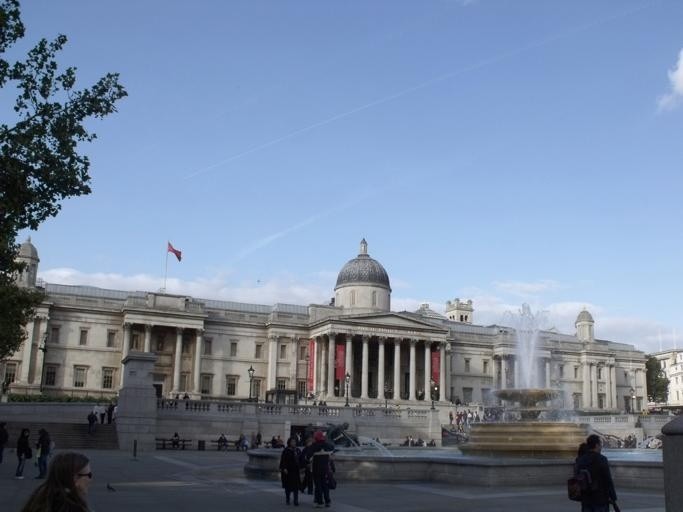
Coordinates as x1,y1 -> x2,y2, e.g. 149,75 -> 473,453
198,440 -> 205,450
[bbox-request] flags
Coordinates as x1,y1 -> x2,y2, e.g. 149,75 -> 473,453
168,242 -> 181,262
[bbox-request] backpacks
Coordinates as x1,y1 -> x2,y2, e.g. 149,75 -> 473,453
568,469 -> 592,502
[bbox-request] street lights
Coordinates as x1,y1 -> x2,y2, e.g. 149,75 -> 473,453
429,376 -> 436,409
628,387 -> 635,413
38,331 -> 49,396
342,369 -> 351,407
303,354 -> 310,404
658,370 -> 667,403
245,364 -> 257,403
504,366 -> 509,388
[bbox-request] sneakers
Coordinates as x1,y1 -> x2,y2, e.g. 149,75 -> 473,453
312,503 -> 324,510
34,475 -> 46,481
14,476 -> 24,480
325,504 -> 331,509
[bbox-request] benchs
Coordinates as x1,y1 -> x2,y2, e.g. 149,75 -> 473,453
155,438 -> 191,450
210,439 -> 236,450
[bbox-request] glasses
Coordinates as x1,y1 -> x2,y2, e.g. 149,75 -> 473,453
77,472 -> 93,480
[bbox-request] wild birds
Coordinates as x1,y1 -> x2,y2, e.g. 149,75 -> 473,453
106,482 -> 115,492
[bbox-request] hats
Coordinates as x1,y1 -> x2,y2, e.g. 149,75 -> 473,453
314,431 -> 326,441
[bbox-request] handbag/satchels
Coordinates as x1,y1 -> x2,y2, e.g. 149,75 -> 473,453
328,470 -> 338,490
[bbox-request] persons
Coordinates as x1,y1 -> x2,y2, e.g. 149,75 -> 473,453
14,429 -> 32,479
170,432 -> 179,449
217,430 -> 337,508
313,401 -> 329,416
0,421 -> 9,463
376,435 -> 436,447
602,434 -> 636,449
21,452 -> 92,512
449,396 -> 504,433
574,434 -> 617,512
174,393 -> 180,407
183,392 -> 191,408
87,401 -> 118,435
35,428 -> 50,479
